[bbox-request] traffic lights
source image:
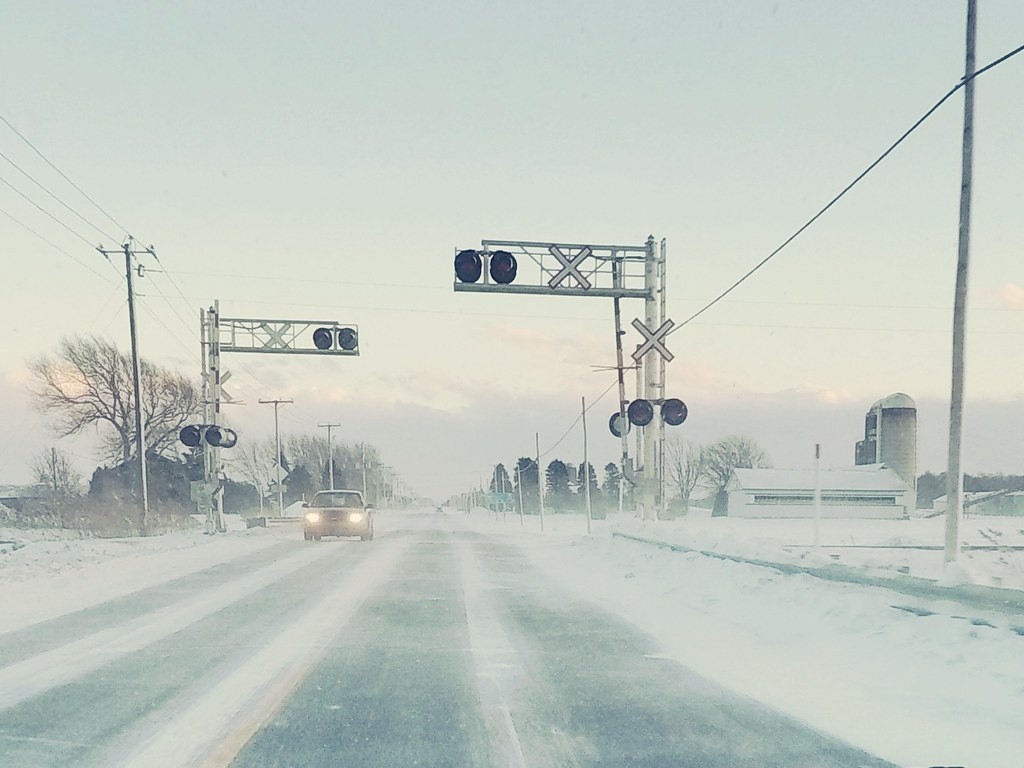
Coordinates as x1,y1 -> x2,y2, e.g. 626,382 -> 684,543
489,251 -> 520,285
205,424 -> 228,447
180,425 -> 203,447
314,327 -> 332,350
339,327 -> 360,350
454,249 -> 484,282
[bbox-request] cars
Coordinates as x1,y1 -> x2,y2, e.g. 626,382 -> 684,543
302,488 -> 375,540
